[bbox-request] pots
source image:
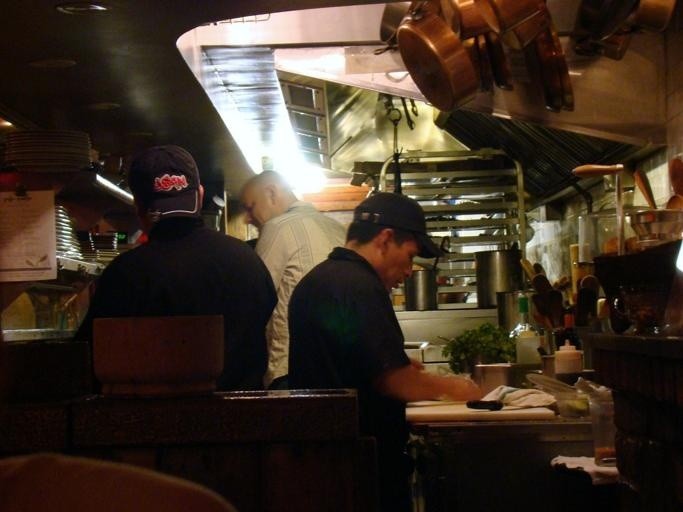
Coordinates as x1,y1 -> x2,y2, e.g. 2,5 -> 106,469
613,284 -> 670,336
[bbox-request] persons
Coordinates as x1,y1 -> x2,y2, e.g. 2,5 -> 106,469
55,143 -> 279,391
287,192 -> 484,510
238,168 -> 347,390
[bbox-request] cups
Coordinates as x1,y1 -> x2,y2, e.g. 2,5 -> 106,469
589,387 -> 616,468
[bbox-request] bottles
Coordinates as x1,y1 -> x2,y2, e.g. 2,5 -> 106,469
508,291 -> 541,364
555,339 -> 583,374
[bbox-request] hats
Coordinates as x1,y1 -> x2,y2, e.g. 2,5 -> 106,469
354,193 -> 445,258
128,144 -> 200,215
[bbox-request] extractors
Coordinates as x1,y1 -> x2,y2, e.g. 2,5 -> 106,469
433,108 -> 667,208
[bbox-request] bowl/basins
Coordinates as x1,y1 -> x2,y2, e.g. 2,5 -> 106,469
555,369 -> 596,385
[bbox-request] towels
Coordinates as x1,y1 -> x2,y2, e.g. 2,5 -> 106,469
480,385 -> 557,407
550,454 -> 622,485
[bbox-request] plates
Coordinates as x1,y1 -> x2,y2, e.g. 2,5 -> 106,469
525,373 -> 575,397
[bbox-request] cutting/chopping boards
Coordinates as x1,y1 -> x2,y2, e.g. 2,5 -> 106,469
404,398 -> 554,422
